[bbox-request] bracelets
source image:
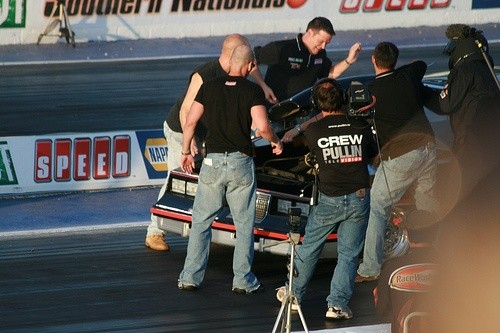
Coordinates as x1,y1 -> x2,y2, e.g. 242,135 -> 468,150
344,58 -> 352,66
181,151 -> 191,155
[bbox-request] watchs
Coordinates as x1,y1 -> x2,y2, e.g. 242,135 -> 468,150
295,123 -> 301,131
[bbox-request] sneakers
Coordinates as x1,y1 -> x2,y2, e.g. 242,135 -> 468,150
277,286 -> 301,309
355,271 -> 380,282
326,305 -> 352,318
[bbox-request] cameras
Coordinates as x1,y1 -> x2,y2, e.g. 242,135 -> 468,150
288,208 -> 302,228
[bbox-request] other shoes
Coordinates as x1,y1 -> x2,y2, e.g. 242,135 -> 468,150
177,278 -> 197,290
234,286 -> 256,294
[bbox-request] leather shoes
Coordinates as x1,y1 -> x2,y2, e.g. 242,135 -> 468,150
145,234 -> 169,251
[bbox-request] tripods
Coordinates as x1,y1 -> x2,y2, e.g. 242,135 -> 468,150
37,0 -> 75,48
262,228 -> 309,333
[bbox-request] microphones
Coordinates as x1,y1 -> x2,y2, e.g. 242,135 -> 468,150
446,24 -> 473,40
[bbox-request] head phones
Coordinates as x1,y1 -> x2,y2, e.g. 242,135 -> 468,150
310,78 -> 348,110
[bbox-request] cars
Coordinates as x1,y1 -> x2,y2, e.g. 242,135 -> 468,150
151,65 -> 498,272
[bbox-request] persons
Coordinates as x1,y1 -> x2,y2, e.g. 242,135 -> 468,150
276,23 -> 500,333
177,44 -> 283,295
145,33 -> 252,251
249,16 -> 362,124
